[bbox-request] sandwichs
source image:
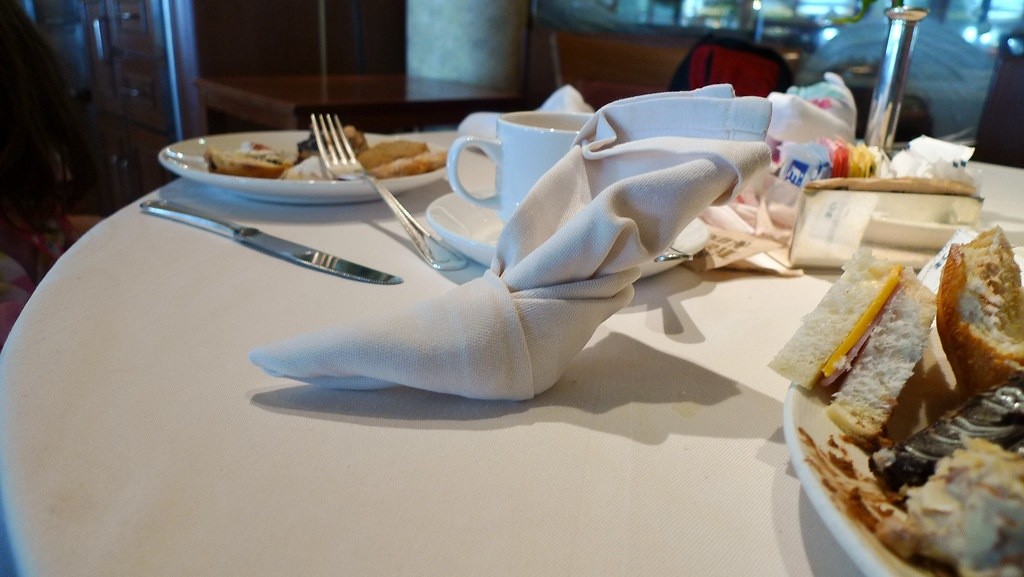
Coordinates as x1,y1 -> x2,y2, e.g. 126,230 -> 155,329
768,255 -> 939,446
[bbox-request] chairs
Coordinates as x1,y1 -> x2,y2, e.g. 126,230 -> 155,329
666,33 -> 795,96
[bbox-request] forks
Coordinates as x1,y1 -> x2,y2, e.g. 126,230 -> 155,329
311,111 -> 471,272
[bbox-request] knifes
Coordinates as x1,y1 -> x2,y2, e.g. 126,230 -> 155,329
140,199 -> 405,286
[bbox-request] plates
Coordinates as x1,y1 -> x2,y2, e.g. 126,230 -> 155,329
782,380 -> 924,577
425,192 -> 711,280
157,129 -> 449,207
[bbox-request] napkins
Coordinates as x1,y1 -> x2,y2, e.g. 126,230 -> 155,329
249,79 -> 774,402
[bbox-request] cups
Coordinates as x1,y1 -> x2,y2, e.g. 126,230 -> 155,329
448,111 -> 601,227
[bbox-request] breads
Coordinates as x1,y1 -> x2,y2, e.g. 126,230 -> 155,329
927,227 -> 1024,392
202,124 -> 448,178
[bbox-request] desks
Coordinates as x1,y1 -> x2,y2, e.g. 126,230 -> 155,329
0,133 -> 1024,577
194,73 -> 525,137
